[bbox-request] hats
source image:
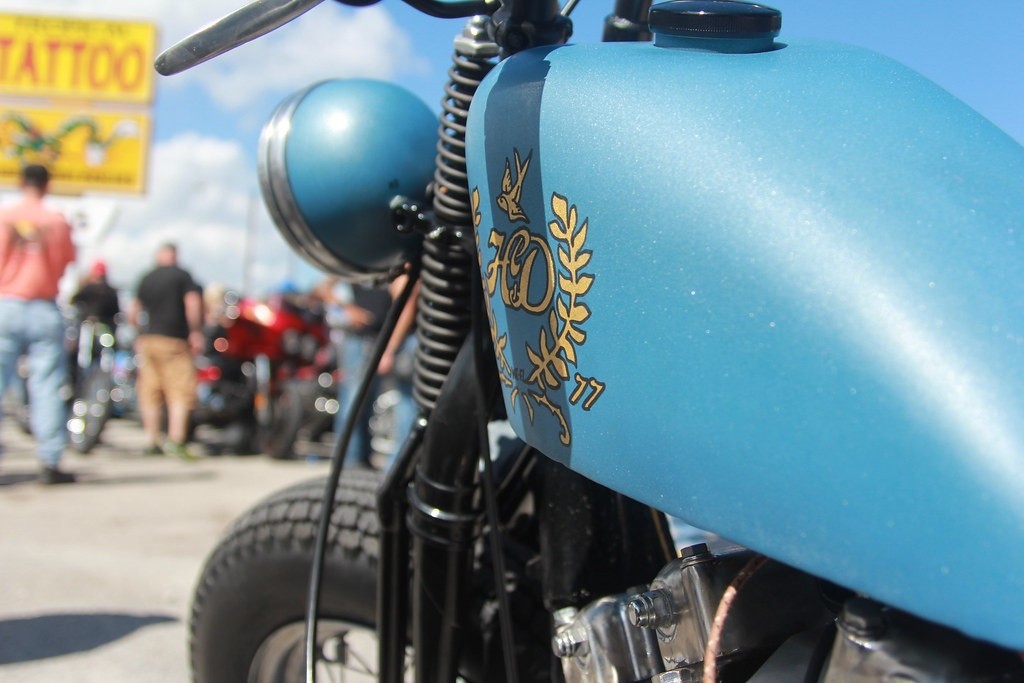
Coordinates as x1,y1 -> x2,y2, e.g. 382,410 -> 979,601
92,261 -> 106,277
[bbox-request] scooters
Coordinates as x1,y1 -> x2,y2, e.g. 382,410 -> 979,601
153,0 -> 1024,683
66,283 -> 344,464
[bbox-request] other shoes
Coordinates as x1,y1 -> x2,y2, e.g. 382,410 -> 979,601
39,467 -> 76,484
162,438 -> 191,458
143,441 -> 164,456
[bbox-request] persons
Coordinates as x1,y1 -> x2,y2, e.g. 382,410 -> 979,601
326,264 -> 419,468
71,260 -> 120,368
0,165 -> 75,484
128,243 -> 205,456
